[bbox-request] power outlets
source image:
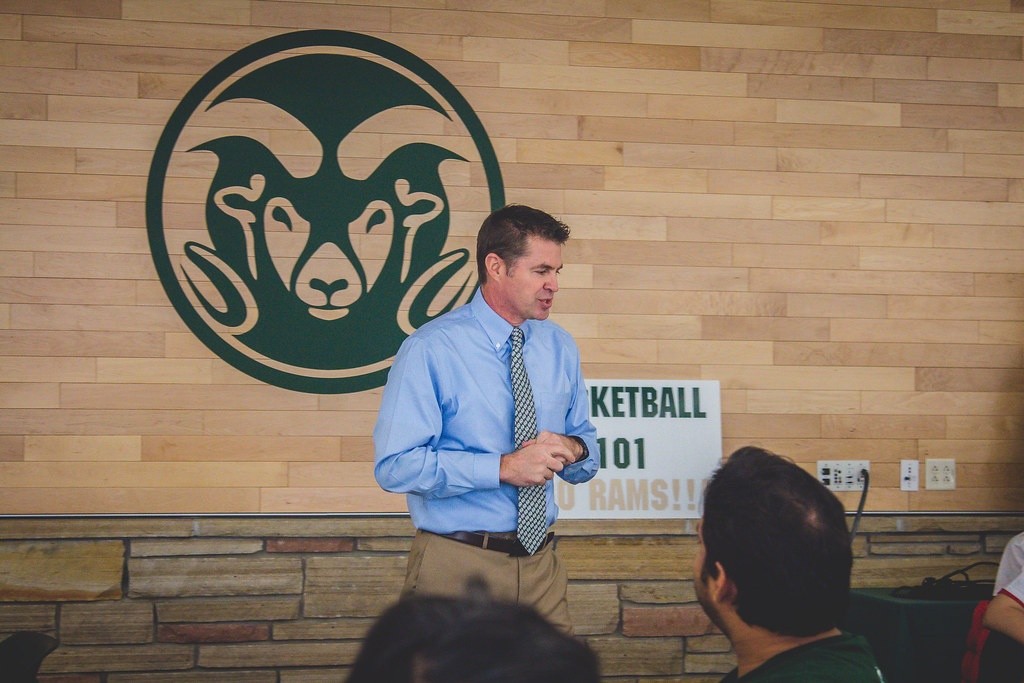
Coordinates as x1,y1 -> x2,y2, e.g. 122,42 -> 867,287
816,461 -> 870,492
899,458 -> 920,493
926,458 -> 957,492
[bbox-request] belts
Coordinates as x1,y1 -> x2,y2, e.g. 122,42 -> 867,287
417,529 -> 554,557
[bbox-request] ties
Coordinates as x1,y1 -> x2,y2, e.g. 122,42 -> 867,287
510,326 -> 547,557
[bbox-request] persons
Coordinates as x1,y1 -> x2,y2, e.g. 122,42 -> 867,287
692,446 -> 886,683
978,531 -> 1024,683
345,593 -> 603,683
372,204 -> 600,635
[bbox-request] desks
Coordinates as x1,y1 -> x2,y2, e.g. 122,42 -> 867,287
852,585 -> 993,683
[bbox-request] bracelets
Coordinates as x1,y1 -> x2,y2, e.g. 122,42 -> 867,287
568,435 -> 588,463
